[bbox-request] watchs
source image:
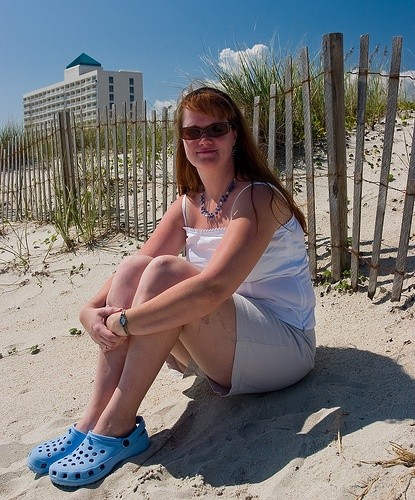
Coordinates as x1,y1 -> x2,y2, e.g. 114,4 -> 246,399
119,309 -> 131,336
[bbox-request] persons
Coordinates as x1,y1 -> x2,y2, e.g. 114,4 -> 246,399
28,87 -> 316,487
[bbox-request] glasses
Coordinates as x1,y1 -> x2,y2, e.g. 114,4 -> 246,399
180,122 -> 233,140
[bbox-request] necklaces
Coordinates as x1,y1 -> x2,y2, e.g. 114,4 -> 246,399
199,179 -> 238,218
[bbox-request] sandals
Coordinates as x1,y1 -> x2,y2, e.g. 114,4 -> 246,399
28,423 -> 89,473
48,416 -> 149,486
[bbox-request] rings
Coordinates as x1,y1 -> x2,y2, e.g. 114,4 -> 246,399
105,345 -> 108,349
